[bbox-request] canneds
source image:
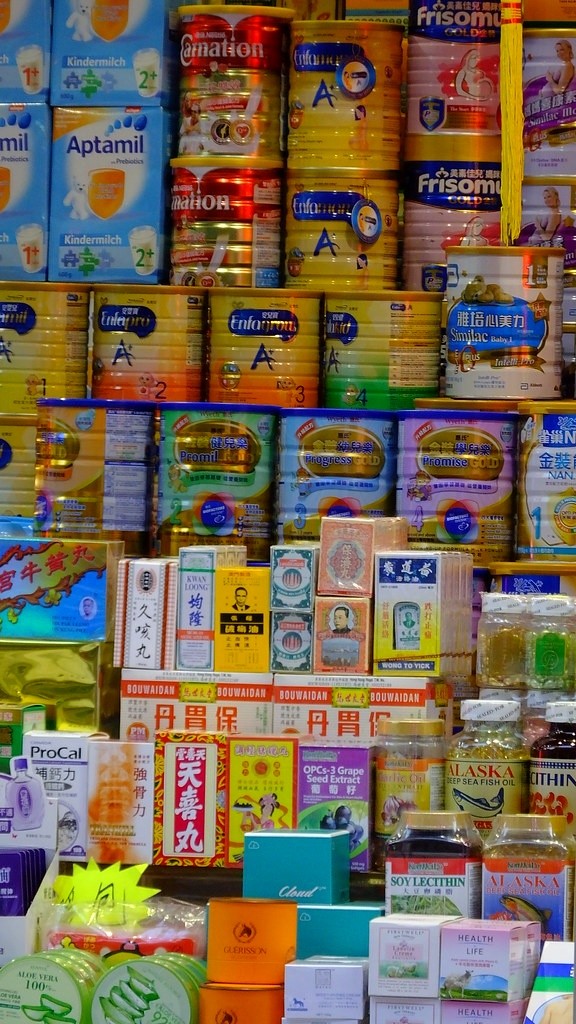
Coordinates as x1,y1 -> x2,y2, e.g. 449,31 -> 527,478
1,1 -> 575,595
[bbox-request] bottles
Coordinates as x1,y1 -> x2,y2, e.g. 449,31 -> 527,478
445,699 -> 529,843
5,758 -> 45,830
529,701 -> 576,843
